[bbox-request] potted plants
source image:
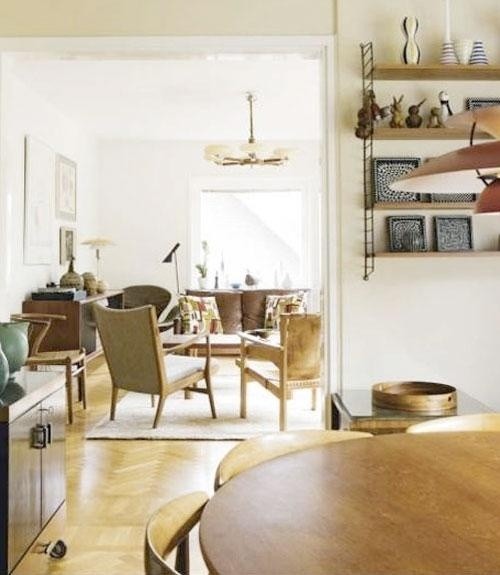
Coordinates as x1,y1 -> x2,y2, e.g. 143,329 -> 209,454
194,240 -> 210,290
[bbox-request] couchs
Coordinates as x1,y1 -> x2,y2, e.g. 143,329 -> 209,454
160,289 -> 312,359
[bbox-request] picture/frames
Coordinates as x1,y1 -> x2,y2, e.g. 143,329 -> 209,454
54,152 -> 78,223
60,225 -> 78,265
23,133 -> 56,266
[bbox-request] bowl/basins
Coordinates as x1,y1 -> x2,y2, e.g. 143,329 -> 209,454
371,379 -> 457,412
230,282 -> 242,288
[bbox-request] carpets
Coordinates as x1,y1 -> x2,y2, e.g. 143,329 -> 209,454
86,354 -> 327,441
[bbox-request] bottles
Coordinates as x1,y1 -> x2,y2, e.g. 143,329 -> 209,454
193,325 -> 198,334
266,313 -> 274,329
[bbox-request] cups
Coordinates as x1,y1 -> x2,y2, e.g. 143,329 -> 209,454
174,317 -> 182,334
210,319 -> 222,334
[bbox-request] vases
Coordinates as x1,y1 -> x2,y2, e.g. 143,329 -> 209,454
59,260 -> 84,293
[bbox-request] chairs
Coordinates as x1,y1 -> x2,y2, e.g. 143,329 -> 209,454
406,413 -> 500,432
9,313 -> 87,424
144,491 -> 209,575
236,313 -> 322,430
213,430 -> 374,490
89,302 -> 216,428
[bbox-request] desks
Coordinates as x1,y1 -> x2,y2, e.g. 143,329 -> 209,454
162,334 -> 281,400
199,432 -> 500,575
330,388 -> 494,434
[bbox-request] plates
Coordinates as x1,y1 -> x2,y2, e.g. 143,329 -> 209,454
373,157 -> 422,203
435,215 -> 474,251
426,156 -> 480,203
466,96 -> 500,111
388,215 -> 428,252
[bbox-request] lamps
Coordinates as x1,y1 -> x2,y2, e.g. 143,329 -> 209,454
80,237 -> 115,279
204,96 -> 296,166
163,242 -> 181,294
386,105 -> 500,220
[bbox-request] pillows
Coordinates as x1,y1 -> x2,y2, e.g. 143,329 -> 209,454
265,295 -> 302,329
178,296 -> 224,334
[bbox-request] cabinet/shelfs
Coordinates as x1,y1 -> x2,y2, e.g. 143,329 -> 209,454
1,384 -> 67,575
360,41 -> 500,281
22,289 -> 125,364
